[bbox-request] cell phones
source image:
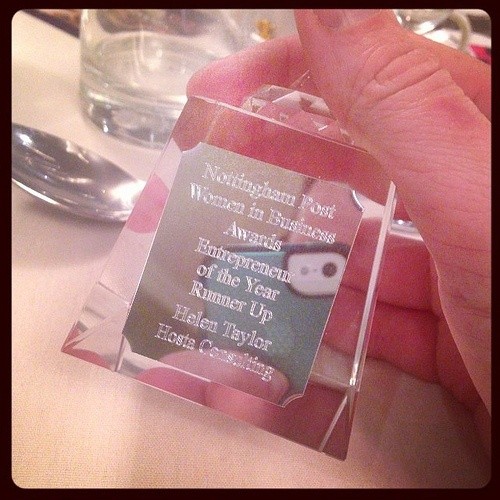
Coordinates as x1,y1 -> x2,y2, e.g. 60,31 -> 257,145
199,242 -> 349,405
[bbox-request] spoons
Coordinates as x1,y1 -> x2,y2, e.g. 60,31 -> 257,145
10,125 -> 137,222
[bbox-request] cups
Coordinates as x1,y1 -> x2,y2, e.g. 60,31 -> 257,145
81,10 -> 256,149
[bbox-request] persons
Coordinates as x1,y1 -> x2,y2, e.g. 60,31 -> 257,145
160,180 -> 361,405
182,9 -> 494,461
